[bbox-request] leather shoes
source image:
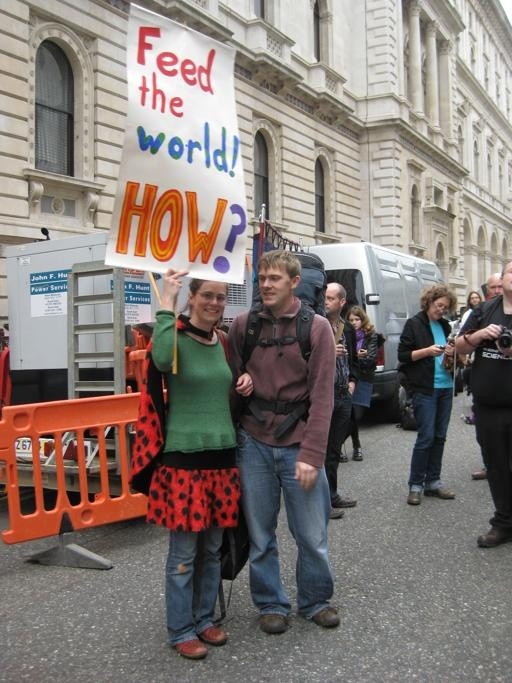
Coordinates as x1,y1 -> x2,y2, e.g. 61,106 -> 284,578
477,526 -> 512,548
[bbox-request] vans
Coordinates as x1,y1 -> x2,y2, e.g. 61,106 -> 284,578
290,241 -> 454,429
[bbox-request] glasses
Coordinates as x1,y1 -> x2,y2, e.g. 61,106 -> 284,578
195,291 -> 227,301
433,301 -> 450,314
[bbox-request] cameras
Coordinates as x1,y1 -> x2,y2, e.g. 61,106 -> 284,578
497,327 -> 512,349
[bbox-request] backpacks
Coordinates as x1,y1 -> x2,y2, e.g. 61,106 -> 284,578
221,502 -> 250,580
239,248 -> 329,440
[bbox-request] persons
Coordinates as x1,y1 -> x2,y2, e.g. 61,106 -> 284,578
320,282 -> 357,520
341,305 -> 378,464
457,261 -> 512,548
151,269 -> 255,660
224,249 -> 340,634
398,284 -> 456,506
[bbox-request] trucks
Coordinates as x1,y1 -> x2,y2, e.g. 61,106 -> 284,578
0,427 -> 139,499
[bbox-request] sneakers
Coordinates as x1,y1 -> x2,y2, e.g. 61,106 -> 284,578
353,448 -> 364,461
328,508 -> 345,518
174,639 -> 209,661
258,613 -> 288,634
407,490 -> 421,506
470,467 -> 487,480
312,606 -> 340,628
200,625 -> 227,647
339,452 -> 349,462
331,493 -> 357,508
423,486 -> 456,500
